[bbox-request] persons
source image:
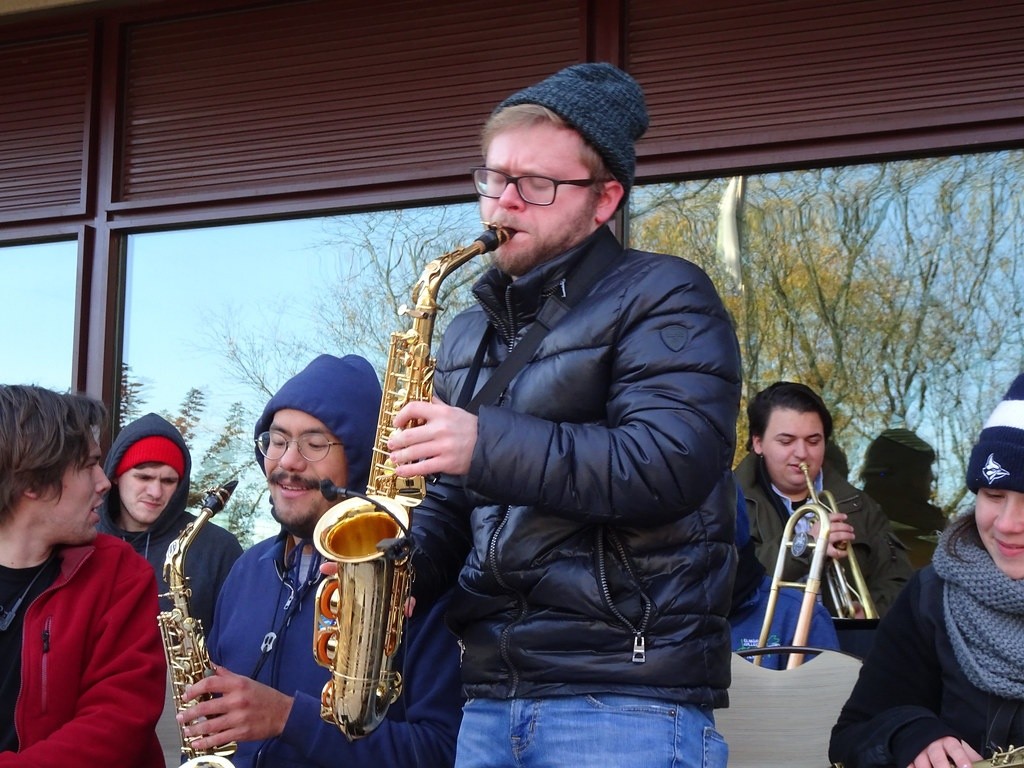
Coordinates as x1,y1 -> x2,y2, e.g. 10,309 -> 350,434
175,353 -> 463,767
0,382 -> 164,767
829,375 -> 1024,768
856,430 -> 949,532
320,60 -> 743,768
97,413 -> 245,646
731,381 -> 888,670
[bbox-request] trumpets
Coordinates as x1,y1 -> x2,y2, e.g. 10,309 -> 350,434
800,461 -> 878,621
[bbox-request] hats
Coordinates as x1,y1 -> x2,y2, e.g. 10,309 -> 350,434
490,63 -> 648,210
966,371 -> 1023,495
115,436 -> 185,479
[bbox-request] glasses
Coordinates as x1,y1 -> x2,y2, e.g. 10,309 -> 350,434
470,164 -> 614,206
254,431 -> 343,462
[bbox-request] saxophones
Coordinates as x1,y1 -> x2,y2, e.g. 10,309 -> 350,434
149,472 -> 241,768
303,223 -> 517,742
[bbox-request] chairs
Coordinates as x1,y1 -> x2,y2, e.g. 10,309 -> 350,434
712,646 -> 867,768
155,665 -> 214,768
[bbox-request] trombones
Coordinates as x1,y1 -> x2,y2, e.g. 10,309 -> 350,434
751,454 -> 884,670
